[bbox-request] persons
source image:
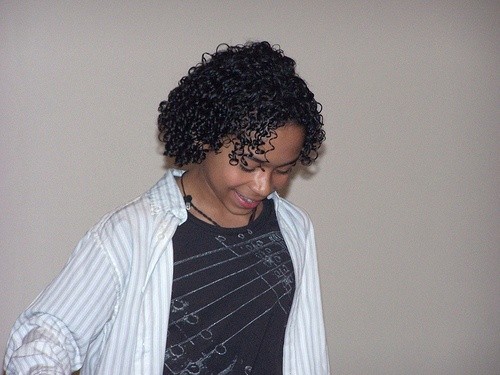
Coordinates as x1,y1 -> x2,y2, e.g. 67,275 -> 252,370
0,39 -> 332,375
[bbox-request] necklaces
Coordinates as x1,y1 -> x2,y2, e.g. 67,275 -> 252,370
180,169 -> 259,230
